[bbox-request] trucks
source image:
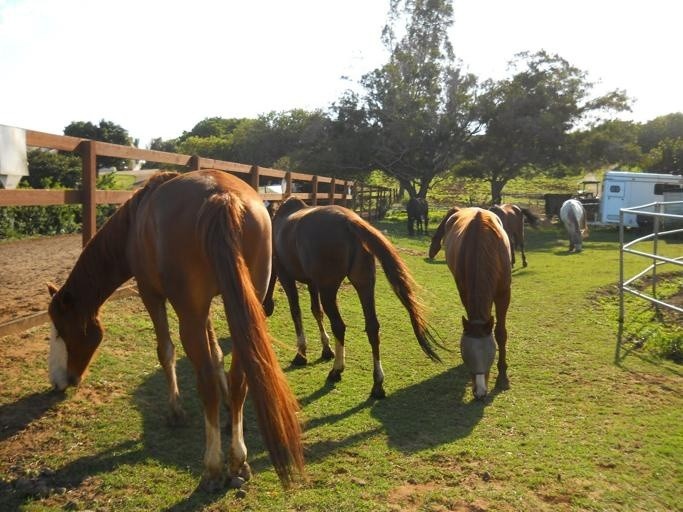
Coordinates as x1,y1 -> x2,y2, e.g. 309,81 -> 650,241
599,170 -> 683,234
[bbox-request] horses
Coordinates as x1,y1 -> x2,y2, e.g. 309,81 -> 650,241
441,206 -> 514,402
428,206 -> 461,261
46,167 -> 304,491
264,194 -> 454,400
487,203 -> 540,268
559,198 -> 587,252
405,197 -> 429,236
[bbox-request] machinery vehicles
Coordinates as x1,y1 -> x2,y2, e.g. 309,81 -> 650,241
544,180 -> 600,222
